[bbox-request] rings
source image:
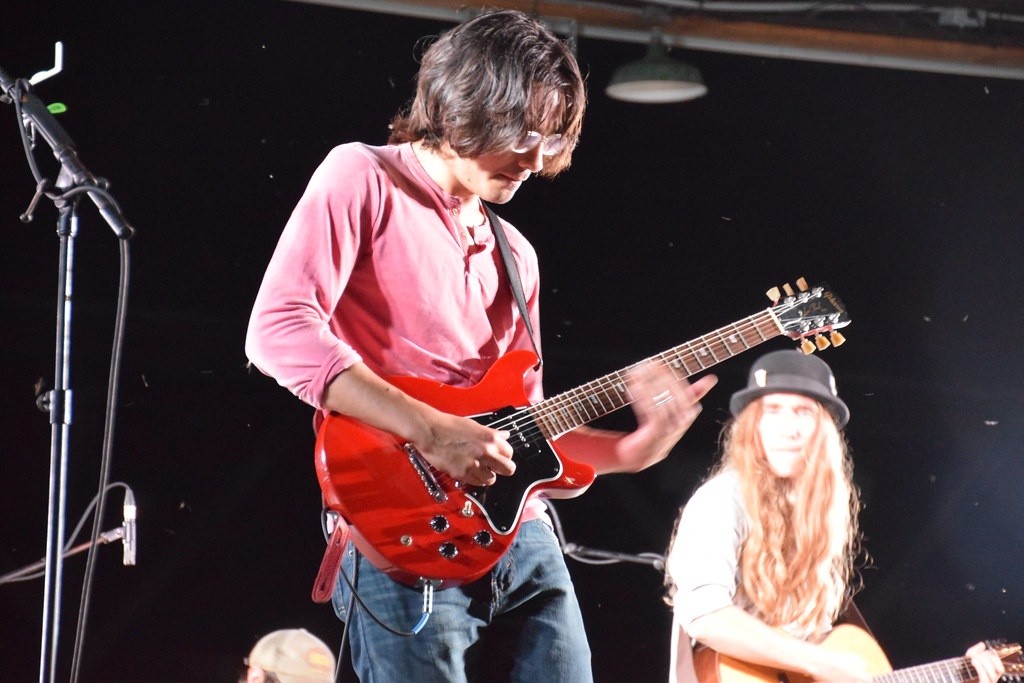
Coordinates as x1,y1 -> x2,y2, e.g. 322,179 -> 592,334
655,391 -> 673,406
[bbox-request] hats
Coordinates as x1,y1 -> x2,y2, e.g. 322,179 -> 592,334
729,348 -> 850,431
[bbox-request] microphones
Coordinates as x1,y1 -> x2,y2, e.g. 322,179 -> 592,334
123,489 -> 137,566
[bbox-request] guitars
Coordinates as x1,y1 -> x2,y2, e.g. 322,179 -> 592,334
313,278 -> 850,591
690,622 -> 1024,683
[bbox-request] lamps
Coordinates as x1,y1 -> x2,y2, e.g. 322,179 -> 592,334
603,22 -> 709,104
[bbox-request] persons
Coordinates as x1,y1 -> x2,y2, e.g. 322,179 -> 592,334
661,349 -> 877,683
243,10 -> 721,683
246,628 -> 335,683
